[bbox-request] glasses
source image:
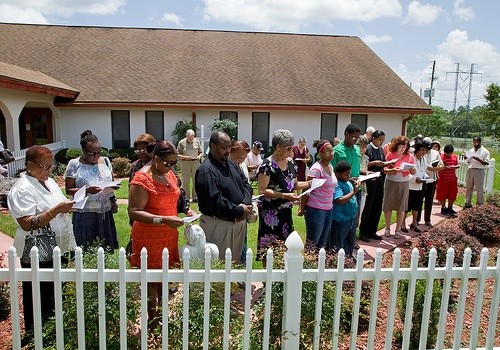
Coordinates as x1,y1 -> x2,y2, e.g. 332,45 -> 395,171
84,149 -> 102,156
32,160 -> 52,171
283,145 -> 293,151
345,132 -> 359,140
158,155 -> 178,167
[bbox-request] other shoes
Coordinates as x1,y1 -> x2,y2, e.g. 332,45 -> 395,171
358,235 -> 369,243
425,222 -> 433,228
371,233 -> 383,240
441,208 -> 450,216
447,207 -> 457,214
385,230 -> 390,236
395,231 -> 401,238
193,198 -> 198,202
401,227 -> 408,233
185,197 -> 189,202
410,224 -> 421,232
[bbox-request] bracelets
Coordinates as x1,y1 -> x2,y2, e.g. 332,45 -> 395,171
153,216 -> 164,226
46,209 -> 56,220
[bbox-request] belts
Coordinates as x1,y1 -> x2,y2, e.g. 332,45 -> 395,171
204,214 -> 245,223
472,168 -> 478,169
182,159 -> 197,161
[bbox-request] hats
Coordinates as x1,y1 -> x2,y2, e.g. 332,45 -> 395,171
253,141 -> 264,151
432,141 -> 440,151
423,137 -> 432,148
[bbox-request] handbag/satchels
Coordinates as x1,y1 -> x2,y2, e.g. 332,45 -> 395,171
110,195 -> 118,213
21,214 -> 56,263
171,169 -> 189,215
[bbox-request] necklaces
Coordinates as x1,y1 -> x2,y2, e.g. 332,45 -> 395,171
150,165 -> 170,189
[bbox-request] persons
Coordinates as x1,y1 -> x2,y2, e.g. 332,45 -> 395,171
436,145 -> 461,217
126,133 -> 197,329
63,129 -> 120,255
463,137 -> 490,210
176,129 -> 203,203
0,140 -> 78,334
200,123 -> 445,296
